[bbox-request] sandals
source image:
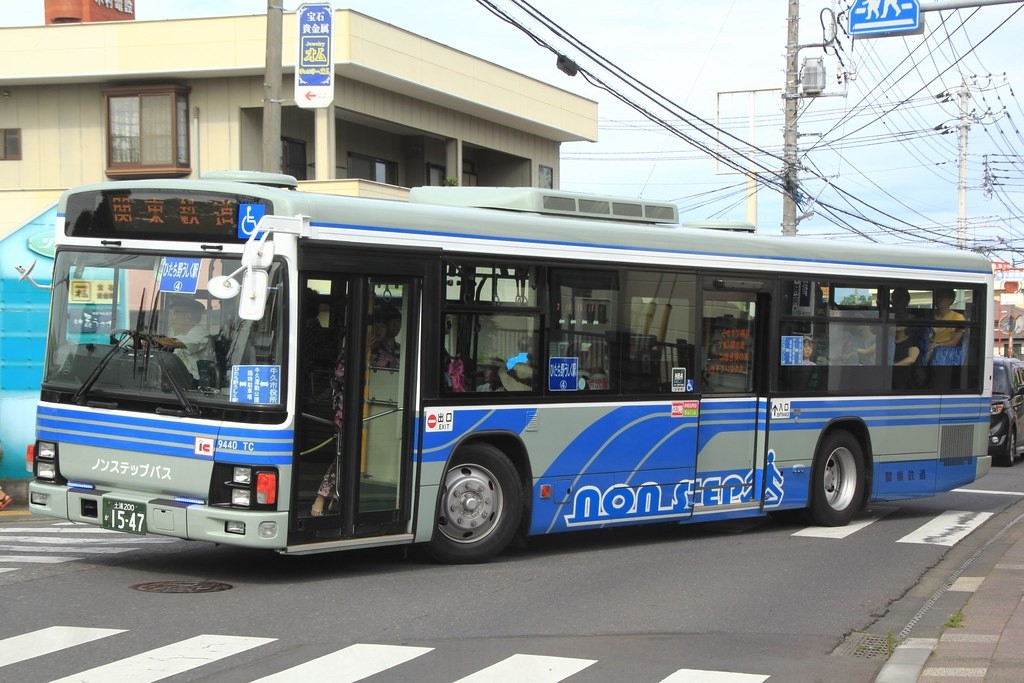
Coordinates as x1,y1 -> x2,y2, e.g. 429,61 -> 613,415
310,505 -> 324,518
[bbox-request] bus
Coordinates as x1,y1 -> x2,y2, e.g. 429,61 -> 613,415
24,172 -> 996,565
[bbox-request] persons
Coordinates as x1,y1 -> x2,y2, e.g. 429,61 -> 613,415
142,296 -> 219,389
802,286 -> 965,366
505,338 -> 530,371
476,358 -> 508,392
311,304 -> 402,516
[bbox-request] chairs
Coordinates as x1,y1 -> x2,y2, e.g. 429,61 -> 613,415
156,283 -> 983,394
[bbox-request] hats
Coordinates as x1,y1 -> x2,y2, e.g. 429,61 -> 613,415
167,293 -> 205,314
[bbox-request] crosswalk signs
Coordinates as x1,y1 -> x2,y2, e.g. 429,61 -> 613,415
847,0 -> 921,36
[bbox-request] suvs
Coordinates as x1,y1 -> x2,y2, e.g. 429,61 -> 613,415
988,356 -> 1024,468
